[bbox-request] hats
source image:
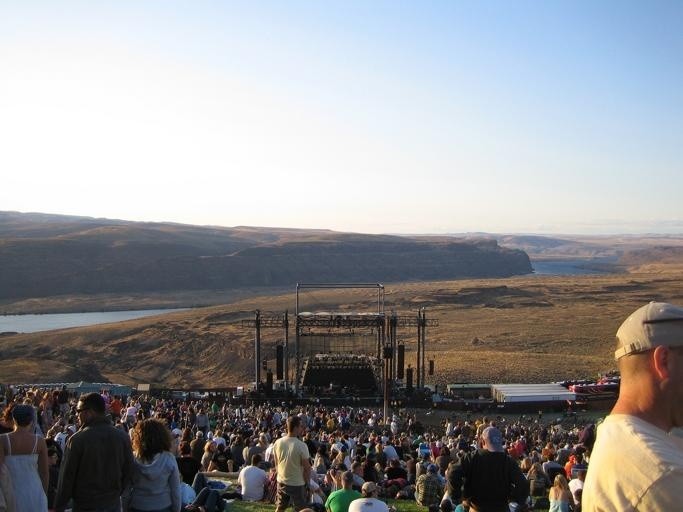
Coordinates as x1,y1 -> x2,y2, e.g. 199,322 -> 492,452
196,431 -> 203,437
435,441 -> 443,448
614,300 -> 683,359
362,481 -> 377,493
207,431 -> 213,438
482,427 -> 504,453
426,464 -> 436,472
171,428 -> 183,437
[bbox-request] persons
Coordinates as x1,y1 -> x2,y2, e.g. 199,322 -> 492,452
1,377 -> 597,511
576,299 -> 683,512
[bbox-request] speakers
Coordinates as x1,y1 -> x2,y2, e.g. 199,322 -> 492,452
277,345 -> 283,379
406,369 -> 413,391
267,374 -> 272,390
397,344 -> 405,379
